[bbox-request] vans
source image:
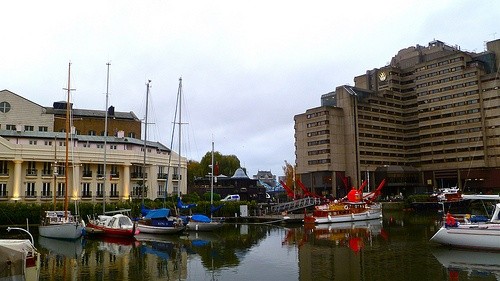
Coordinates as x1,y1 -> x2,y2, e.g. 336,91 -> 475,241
220,195 -> 240,202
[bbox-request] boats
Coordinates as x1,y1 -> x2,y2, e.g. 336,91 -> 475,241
430,194 -> 500,249
313,178 -> 385,223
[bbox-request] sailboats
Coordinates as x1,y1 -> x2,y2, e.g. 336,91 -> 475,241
38,62 -> 86,240
132,77 -> 227,236
86,62 -> 140,238
